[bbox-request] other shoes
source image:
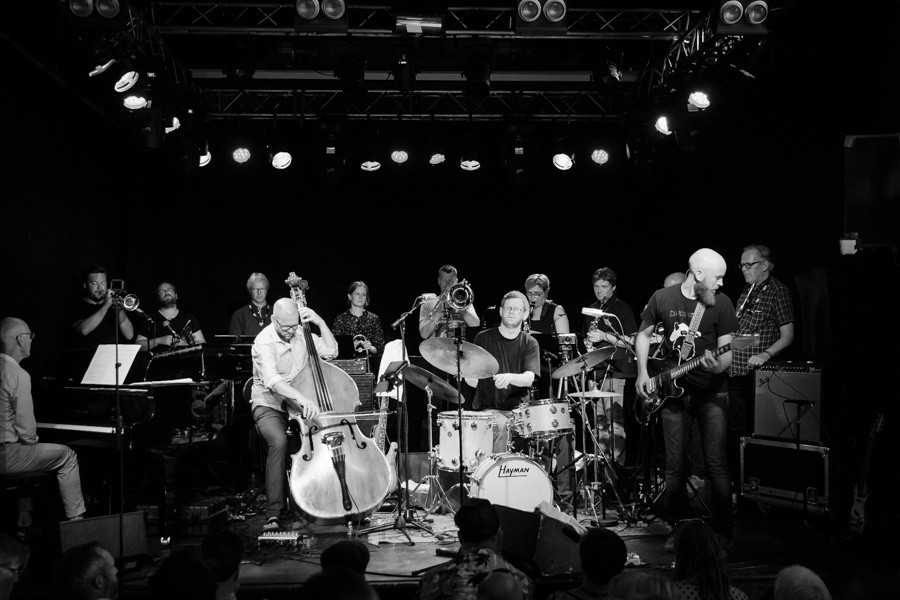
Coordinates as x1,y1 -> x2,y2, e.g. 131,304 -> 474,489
262,516 -> 279,530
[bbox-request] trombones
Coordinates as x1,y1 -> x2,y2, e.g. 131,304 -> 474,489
429,279 -> 475,325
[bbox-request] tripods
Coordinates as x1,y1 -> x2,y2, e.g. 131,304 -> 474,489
546,345 -> 713,528
354,300 -> 456,547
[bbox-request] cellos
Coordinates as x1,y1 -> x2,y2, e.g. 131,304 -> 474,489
284,272 -> 394,527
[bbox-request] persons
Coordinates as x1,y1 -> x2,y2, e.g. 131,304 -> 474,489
582,268 -> 637,491
0,317 -> 86,521
249,298 -> 338,531
134,281 -> 206,351
0,498 -> 832,600
525,274 -> 571,496
726,244 -> 794,437
332,281 -> 385,354
419,265 -> 480,339
464,291 -> 541,455
67,262 -> 134,344
229,272 -> 273,335
635,248 -> 741,536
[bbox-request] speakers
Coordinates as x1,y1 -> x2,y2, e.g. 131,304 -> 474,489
754,361 -> 822,443
491,504 -> 583,575
29,510 -> 150,570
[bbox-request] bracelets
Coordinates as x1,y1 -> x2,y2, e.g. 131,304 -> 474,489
764,351 -> 772,358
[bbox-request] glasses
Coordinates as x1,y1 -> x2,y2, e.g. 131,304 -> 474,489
526,290 -> 543,298
738,261 -> 760,269
276,320 -> 298,331
21,332 -> 35,339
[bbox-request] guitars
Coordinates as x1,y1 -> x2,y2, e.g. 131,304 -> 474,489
376,381 -> 401,497
637,332 -> 760,414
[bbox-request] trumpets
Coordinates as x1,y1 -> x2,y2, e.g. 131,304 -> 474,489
102,289 -> 139,311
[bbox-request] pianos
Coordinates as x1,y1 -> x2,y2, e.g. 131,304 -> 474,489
28,372 -> 211,520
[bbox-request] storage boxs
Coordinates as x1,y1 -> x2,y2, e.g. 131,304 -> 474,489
740,436 -> 840,505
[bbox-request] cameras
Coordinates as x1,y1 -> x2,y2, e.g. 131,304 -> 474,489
559,332 -> 577,344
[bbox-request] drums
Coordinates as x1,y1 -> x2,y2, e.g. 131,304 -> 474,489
437,396 -> 571,514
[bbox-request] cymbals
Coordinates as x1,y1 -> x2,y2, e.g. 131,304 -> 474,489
401,362 -> 466,405
551,345 -> 617,379
568,387 -> 622,399
419,336 -> 501,380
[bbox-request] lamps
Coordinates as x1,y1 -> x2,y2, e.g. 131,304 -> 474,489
291,0 -> 351,32
515,0 -> 569,36
716,0 -> 769,36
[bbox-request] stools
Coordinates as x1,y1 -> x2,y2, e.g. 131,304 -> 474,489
244,377 -> 305,528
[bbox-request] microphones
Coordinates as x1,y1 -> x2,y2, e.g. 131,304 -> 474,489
136,308 -> 155,324
163,321 -> 179,338
783,399 -> 815,407
176,319 -> 192,339
581,307 -> 615,318
